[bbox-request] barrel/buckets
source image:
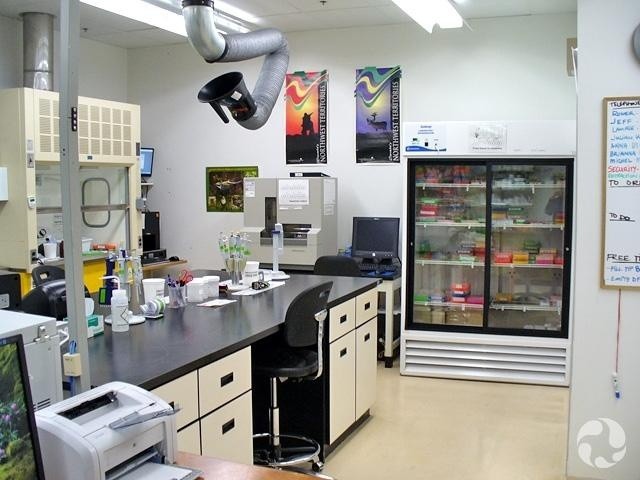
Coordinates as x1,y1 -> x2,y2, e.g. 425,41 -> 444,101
43,243 -> 58,258
240,260 -> 260,287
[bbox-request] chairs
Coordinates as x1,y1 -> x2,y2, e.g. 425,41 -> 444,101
251,280 -> 336,473
31,266 -> 91,294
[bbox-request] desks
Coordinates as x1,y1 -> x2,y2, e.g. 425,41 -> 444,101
176,449 -> 330,480
63,269 -> 384,466
258,262 -> 402,368
143,258 -> 188,272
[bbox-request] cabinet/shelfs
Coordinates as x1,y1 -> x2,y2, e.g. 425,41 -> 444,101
402,153 -> 574,390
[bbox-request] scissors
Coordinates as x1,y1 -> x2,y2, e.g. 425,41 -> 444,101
178,270 -> 193,285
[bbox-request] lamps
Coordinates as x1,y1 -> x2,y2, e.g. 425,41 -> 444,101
78,1 -> 252,41
391,0 -> 463,33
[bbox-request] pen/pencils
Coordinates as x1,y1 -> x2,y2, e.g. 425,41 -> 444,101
166,274 -> 185,307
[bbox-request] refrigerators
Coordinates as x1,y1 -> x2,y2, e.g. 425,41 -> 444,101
401,119 -> 573,340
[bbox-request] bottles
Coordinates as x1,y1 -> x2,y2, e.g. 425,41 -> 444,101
111,290 -> 130,333
85,297 -> 95,317
105,252 -> 143,287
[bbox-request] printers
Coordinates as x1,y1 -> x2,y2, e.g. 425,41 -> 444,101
33,380 -> 203,480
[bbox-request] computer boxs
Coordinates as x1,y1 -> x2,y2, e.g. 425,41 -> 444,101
143,248 -> 166,259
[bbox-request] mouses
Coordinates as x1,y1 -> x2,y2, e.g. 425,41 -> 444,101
375,268 -> 386,276
169,256 -> 179,261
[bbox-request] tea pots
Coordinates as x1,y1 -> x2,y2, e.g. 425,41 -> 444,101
241,271 -> 270,288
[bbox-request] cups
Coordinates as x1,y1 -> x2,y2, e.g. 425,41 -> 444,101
42,244 -> 58,260
189,274 -> 220,303
245,260 -> 258,272
169,286 -> 187,309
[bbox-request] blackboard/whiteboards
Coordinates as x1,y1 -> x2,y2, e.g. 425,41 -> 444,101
599,96 -> 640,292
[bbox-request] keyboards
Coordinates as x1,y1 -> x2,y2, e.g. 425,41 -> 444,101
356,263 -> 398,272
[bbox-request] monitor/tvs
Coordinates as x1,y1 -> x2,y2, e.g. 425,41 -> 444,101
0,334 -> 46,480
352,217 -> 399,263
142,211 -> 160,251
140,147 -> 154,182
